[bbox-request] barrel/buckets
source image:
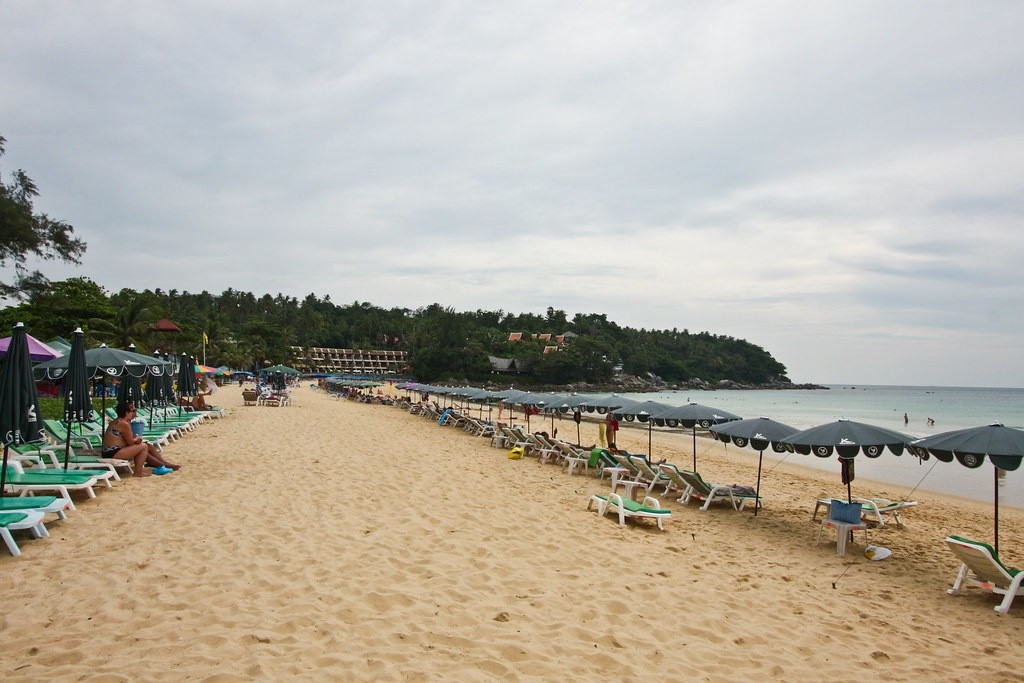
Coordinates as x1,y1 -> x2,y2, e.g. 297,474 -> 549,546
132,423 -> 145,437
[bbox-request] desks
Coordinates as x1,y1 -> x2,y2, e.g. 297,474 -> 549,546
819,520 -> 868,556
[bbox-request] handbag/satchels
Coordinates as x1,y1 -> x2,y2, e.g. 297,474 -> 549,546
508,447 -> 523,459
864,544 -> 893,561
131,420 -> 144,438
831,499 -> 861,523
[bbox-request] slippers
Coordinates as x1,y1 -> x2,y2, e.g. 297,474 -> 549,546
152,465 -> 173,475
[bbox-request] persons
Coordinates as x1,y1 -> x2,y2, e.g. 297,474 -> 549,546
179,389 -> 213,411
497,400 -> 668,469
127,404 -> 179,471
102,402 -> 151,477
214,375 -> 295,389
904,414 -> 909,425
314,380 -> 453,419
927,418 -> 935,426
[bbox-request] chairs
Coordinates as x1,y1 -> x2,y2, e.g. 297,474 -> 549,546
812,496 -> 917,523
0,396 -> 225,559
244,382 -> 292,407
318,367 -> 763,530
946,534 -> 1024,614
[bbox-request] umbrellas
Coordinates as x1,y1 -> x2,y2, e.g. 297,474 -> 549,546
326,377 -> 385,389
648,402 -> 743,473
708,417 -> 802,516
0,322 -> 197,495
194,365 -> 299,379
780,418 -> 919,545
905,420 -> 1024,555
394,381 -> 640,448
